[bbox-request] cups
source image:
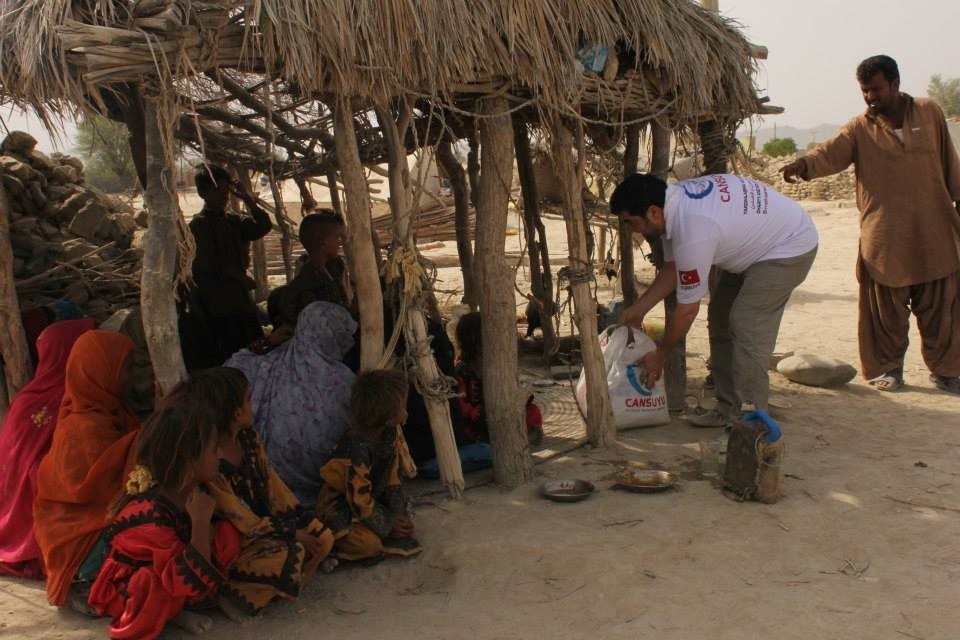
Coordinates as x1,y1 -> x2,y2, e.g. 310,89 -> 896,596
700,438 -> 721,477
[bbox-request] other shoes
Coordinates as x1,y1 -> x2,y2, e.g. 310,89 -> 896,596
689,405 -> 734,427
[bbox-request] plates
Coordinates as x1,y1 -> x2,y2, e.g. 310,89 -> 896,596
539,477 -> 597,501
611,469 -> 676,492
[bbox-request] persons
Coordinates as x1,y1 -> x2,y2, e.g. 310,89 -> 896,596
223,302 -> 361,499
246,284 -> 307,360
69,389 -> 237,637
186,170 -> 454,386
450,315 -> 543,453
608,172 -> 820,432
779,55 -> 960,399
0,321 -> 96,573
189,367 -> 334,622
37,331 -> 144,614
314,371 -> 411,571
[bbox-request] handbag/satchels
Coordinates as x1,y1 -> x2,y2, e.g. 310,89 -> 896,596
576,322 -> 674,431
725,409 -> 784,502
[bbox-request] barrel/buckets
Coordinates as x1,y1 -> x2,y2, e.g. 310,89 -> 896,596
722,407 -> 786,505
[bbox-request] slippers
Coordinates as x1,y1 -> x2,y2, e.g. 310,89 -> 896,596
875,373 -> 905,391
936,378 -> 960,395
